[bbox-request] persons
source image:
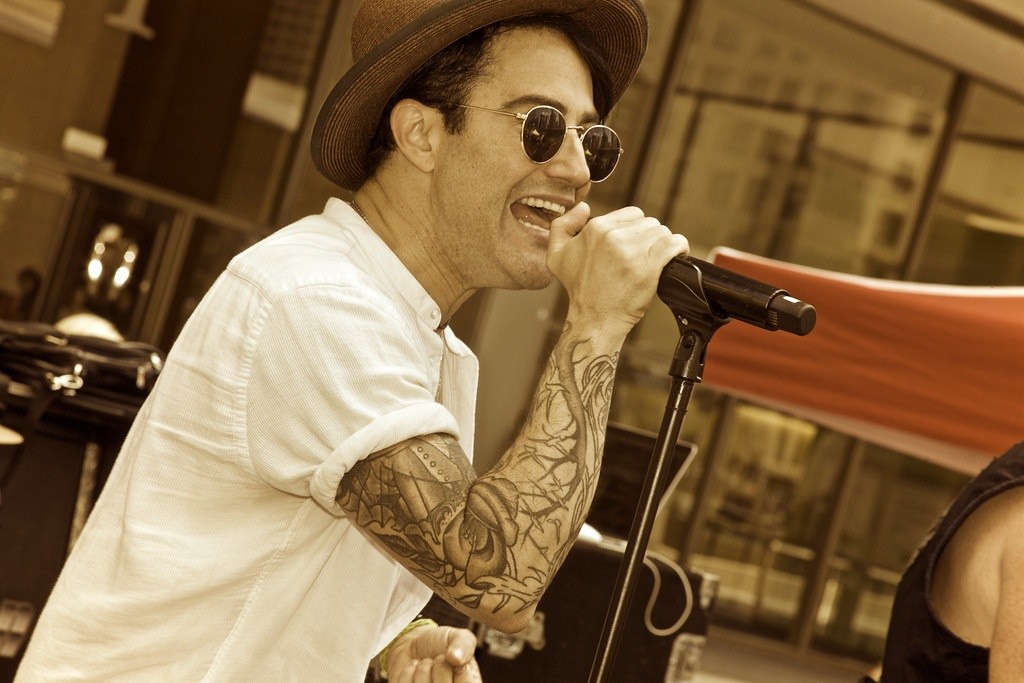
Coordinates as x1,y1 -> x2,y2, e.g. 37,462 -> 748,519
12,0 -> 689,683
881,440 -> 1024,683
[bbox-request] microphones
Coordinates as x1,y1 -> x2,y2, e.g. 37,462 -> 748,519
657,254 -> 817,336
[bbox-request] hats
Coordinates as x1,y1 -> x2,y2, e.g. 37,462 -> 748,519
308,0 -> 649,192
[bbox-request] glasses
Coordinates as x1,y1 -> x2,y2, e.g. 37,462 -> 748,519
436,104 -> 623,184
